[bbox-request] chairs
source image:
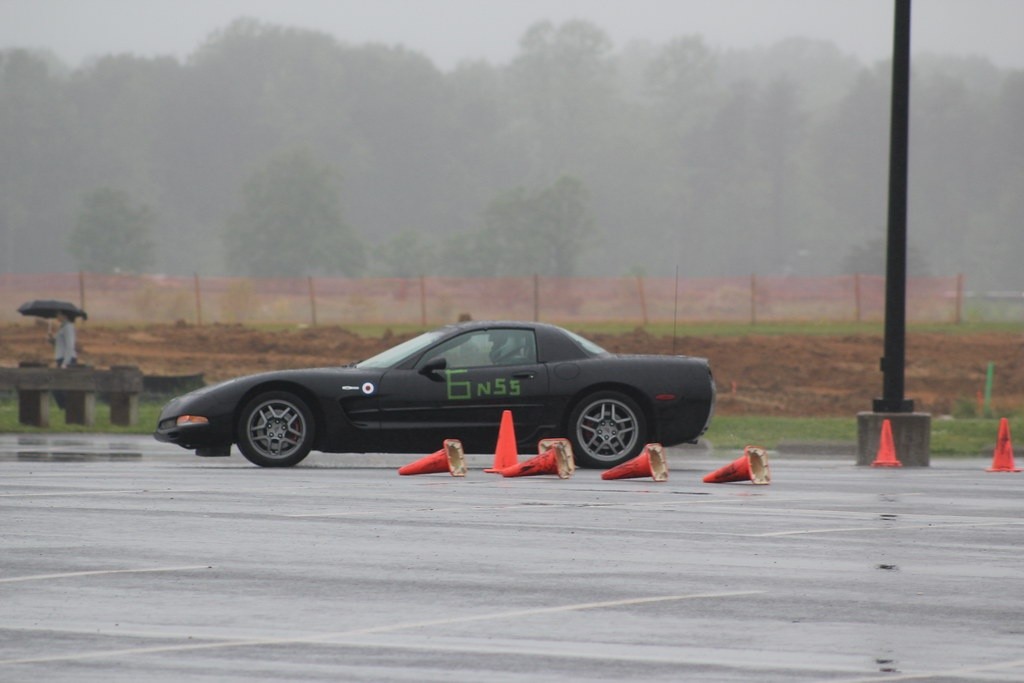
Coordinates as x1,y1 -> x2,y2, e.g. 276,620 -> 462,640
524,333 -> 535,364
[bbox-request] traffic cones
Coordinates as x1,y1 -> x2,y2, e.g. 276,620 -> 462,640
600,442 -> 670,484
499,442 -> 570,480
483,409 -> 518,472
540,437 -> 576,473
398,438 -> 469,478
702,444 -> 772,485
985,417 -> 1023,473
871,417 -> 904,467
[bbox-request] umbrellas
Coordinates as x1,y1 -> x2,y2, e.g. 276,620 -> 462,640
16,300 -> 87,338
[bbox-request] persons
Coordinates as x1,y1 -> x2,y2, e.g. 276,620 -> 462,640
488,330 -> 525,366
47,309 -> 77,409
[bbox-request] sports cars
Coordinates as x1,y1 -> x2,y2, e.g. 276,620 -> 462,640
153,320 -> 717,468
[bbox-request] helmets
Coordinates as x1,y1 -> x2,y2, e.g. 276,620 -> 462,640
488,333 -> 526,363
